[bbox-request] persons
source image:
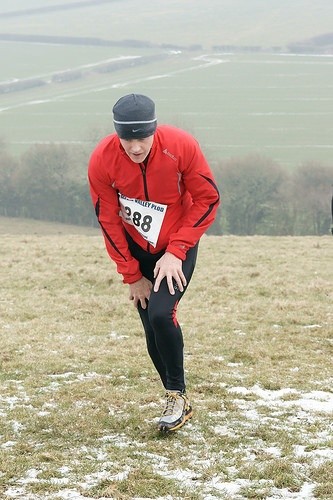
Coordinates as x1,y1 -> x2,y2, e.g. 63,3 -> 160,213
85,92 -> 219,432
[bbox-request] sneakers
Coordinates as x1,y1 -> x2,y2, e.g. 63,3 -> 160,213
157,388 -> 193,432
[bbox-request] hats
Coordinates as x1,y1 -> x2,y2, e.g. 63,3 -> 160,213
111,93 -> 157,140
